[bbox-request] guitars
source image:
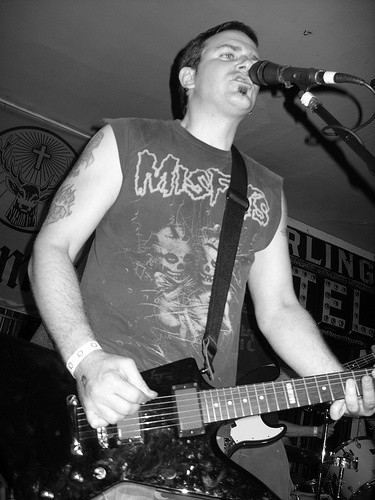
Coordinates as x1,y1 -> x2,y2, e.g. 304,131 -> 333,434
24,357 -> 375,500
216,351 -> 375,459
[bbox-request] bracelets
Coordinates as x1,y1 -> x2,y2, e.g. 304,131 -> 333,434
66,341 -> 102,378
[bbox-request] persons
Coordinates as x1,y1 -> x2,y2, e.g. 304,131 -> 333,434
25,22 -> 375,500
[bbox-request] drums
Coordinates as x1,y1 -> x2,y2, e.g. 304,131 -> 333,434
322,436 -> 375,500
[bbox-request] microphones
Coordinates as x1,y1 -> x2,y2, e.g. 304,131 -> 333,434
248,59 -> 365,86
